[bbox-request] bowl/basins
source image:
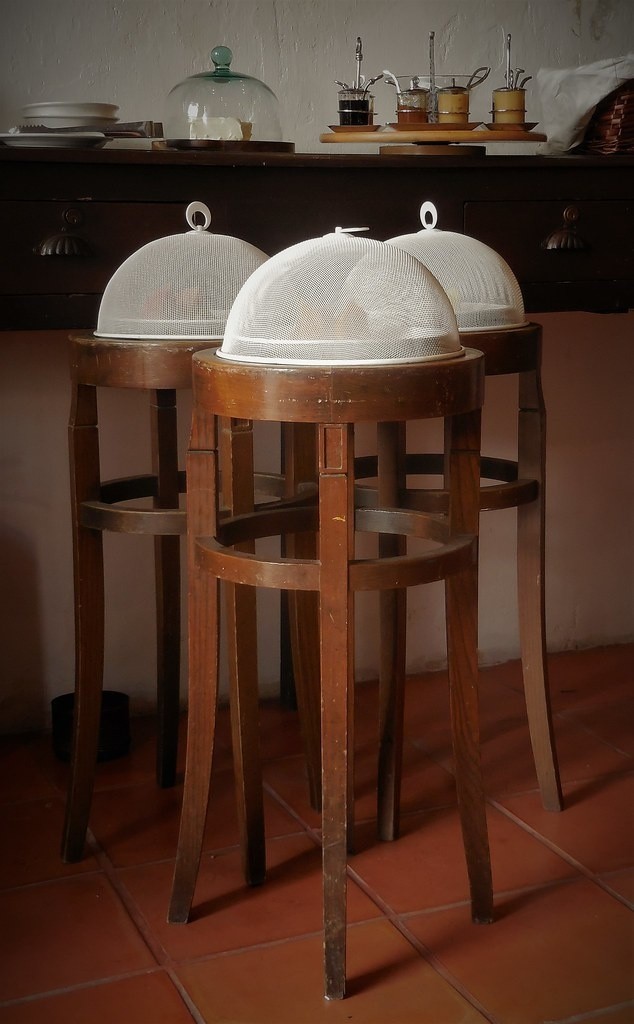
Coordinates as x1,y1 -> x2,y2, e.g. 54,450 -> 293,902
21,101 -> 121,117
23,116 -> 121,128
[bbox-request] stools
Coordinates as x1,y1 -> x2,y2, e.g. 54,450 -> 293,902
280,316 -> 565,842
61,326 -> 321,889
168,345 -> 496,1001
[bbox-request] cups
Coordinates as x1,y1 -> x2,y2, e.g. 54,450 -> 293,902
491,90 -> 527,124
369,95 -> 376,126
436,89 -> 469,124
337,90 -> 370,126
397,91 -> 431,123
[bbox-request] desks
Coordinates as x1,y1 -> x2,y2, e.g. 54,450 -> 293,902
0,146 -> 633,331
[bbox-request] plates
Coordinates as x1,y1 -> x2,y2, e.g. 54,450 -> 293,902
483,120 -> 541,132
0,133 -> 106,150
327,124 -> 380,132
385,120 -> 485,132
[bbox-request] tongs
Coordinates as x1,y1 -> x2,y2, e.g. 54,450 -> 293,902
16,120 -> 156,138
